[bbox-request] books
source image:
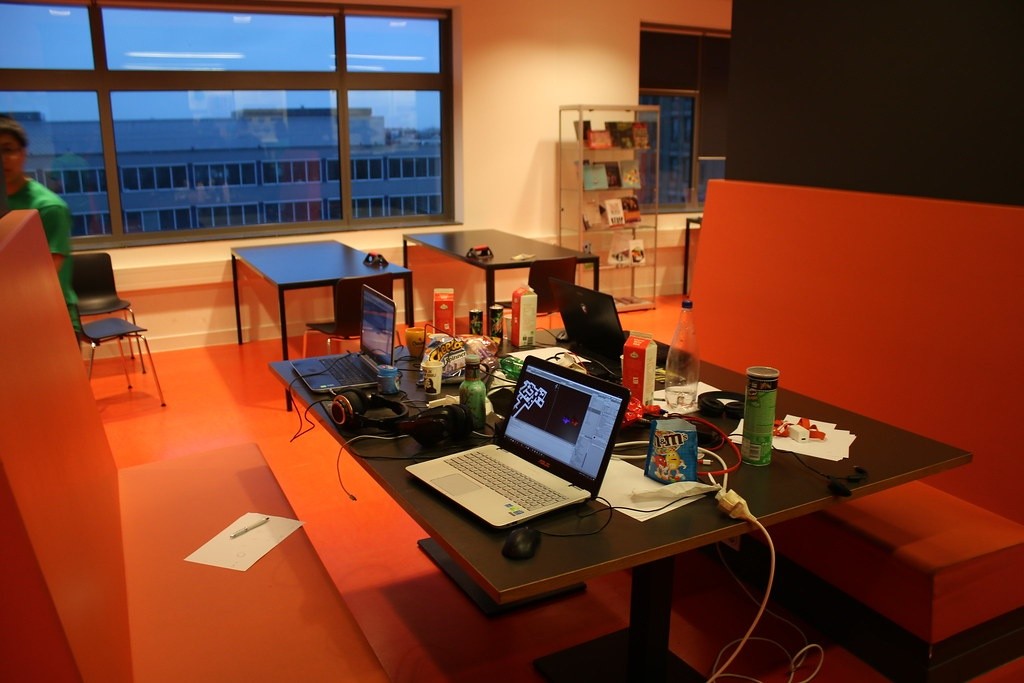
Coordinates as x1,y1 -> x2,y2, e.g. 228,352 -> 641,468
575,120 -> 649,267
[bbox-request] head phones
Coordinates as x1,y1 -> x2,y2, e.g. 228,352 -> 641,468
331,386 -> 408,430
395,403 -> 473,447
697,391 -> 747,420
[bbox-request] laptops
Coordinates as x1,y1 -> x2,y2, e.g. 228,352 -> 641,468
548,276 -> 690,366
404,356 -> 633,528
289,284 -> 397,392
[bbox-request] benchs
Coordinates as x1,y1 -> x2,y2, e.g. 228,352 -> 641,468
0,206 -> 391,683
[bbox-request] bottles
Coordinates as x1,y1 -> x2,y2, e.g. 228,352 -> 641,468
459,354 -> 486,431
665,300 -> 700,409
377,364 -> 399,394
498,356 -> 524,377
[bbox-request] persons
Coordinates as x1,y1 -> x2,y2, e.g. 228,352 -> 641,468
0,116 -> 79,345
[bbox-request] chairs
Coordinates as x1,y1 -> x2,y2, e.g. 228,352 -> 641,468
75,304 -> 166,407
70,253 -> 147,373
303,272 -> 395,358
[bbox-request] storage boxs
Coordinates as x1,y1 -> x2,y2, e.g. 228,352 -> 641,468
433,288 -> 455,337
510,284 -> 537,347
622,330 -> 657,407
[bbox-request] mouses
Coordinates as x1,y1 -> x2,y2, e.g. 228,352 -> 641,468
556,330 -> 570,343
502,527 -> 541,562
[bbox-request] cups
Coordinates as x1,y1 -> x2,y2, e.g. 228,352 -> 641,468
505,316 -> 512,341
406,327 -> 427,356
421,361 -> 444,396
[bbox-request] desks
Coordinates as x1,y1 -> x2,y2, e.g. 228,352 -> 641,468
402,229 -> 599,337
230,240 -> 414,411
268,328 -> 973,683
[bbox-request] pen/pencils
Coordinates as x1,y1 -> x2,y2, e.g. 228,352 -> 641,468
229,517 -> 270,539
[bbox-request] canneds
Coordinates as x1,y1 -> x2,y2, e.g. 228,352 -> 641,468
469,309 -> 483,336
741,366 -> 780,466
489,304 -> 504,346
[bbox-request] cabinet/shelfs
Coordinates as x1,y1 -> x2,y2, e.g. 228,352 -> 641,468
556,104 -> 660,311
678,178 -> 1024,683
